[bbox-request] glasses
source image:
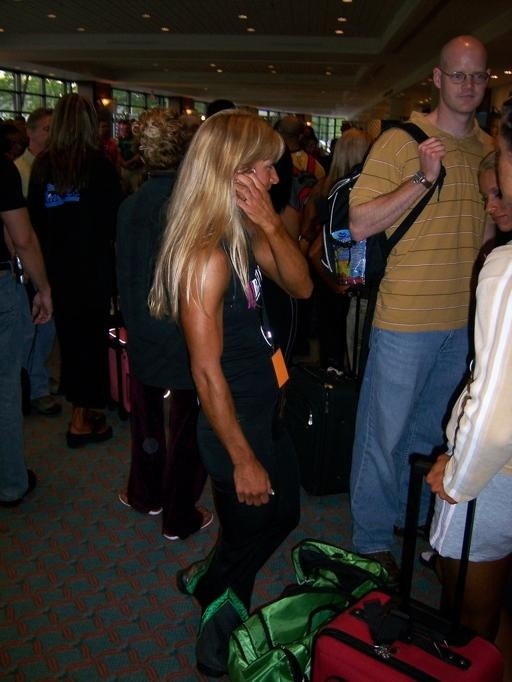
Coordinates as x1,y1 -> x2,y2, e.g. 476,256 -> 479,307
440,66 -> 489,86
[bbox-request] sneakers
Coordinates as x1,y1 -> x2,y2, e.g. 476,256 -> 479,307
358,549 -> 440,597
20,395 -> 63,416
175,565 -> 192,595
0,468 -> 38,509
195,659 -> 226,679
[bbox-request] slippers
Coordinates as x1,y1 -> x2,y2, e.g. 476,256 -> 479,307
160,506 -> 213,542
65,410 -> 113,449
117,489 -> 163,516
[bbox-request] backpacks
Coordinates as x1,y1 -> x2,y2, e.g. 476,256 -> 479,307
314,121 -> 446,303
290,152 -> 321,239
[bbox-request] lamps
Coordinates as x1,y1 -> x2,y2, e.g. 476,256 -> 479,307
182,105 -> 198,116
304,119 -> 312,127
96,96 -> 113,110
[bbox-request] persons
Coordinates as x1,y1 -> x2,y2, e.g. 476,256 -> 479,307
1,93 -> 376,540
348,35 -> 511,643
145,108 -> 313,612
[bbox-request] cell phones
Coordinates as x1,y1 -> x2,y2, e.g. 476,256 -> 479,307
15,256 -> 24,285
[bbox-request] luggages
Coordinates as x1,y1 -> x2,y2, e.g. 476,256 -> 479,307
307,446 -> 507,682
279,356 -> 356,499
106,296 -> 135,420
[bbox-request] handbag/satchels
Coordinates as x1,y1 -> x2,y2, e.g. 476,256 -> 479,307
224,534 -> 393,682
